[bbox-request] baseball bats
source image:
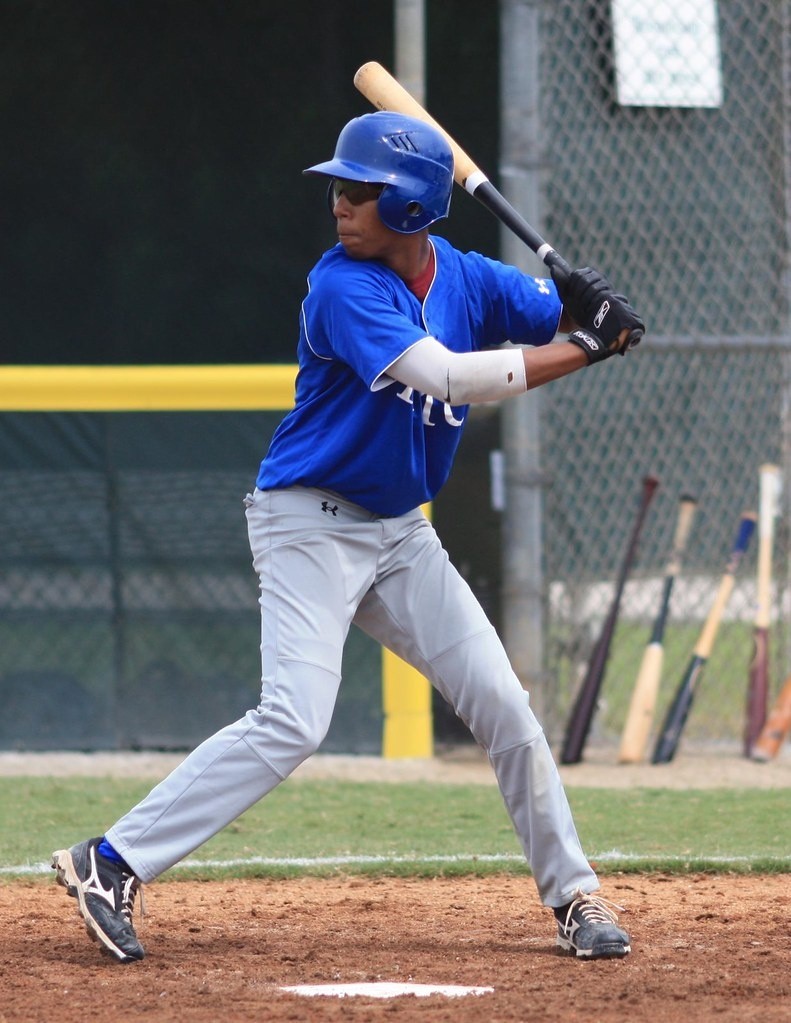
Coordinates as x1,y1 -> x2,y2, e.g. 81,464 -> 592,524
650,512 -> 753,765
750,676 -> 791,757
560,475 -> 656,759
744,464 -> 783,750
614,496 -> 700,759
354,58 -> 643,350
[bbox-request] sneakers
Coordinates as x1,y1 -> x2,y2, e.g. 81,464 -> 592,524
555,887 -> 632,960
51,835 -> 148,961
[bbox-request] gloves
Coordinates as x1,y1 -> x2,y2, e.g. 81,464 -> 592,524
567,295 -> 647,366
550,265 -> 630,329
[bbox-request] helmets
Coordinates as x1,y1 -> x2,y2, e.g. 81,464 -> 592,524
302,111 -> 455,235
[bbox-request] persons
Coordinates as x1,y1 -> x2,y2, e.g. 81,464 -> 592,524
51,113 -> 648,967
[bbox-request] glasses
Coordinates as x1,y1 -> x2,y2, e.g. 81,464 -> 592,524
332,177 -> 383,206
